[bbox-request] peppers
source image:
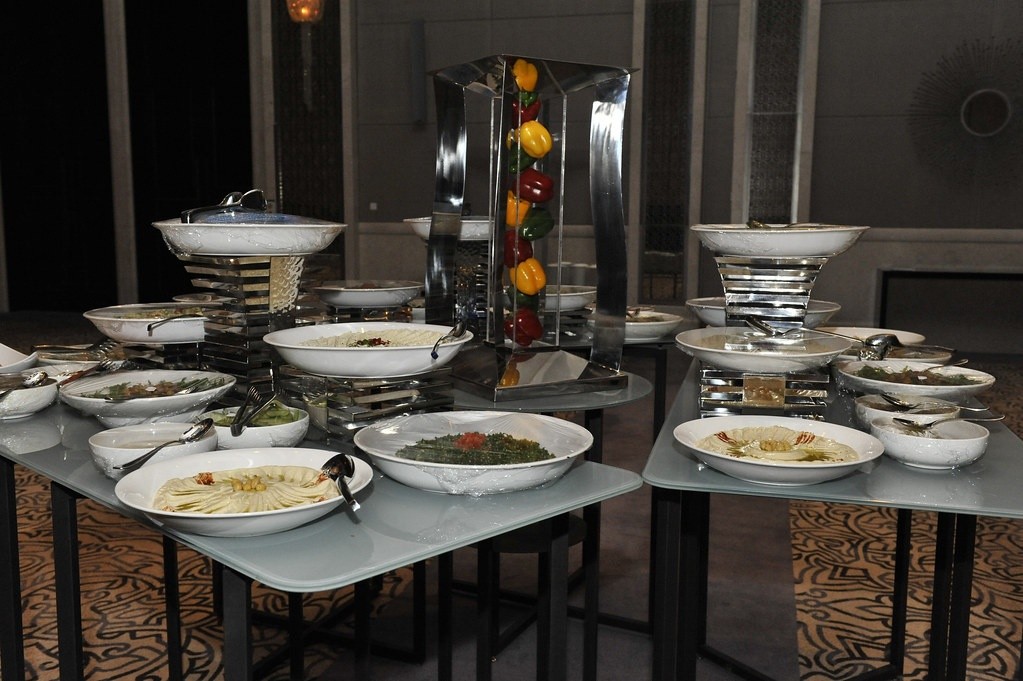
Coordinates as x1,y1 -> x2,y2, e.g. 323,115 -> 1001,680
496,60 -> 555,388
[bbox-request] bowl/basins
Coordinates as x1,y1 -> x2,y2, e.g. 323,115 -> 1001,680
201,405 -> 310,450
88,422 -> 218,481
853,390 -> 960,430
868,416 -> 990,469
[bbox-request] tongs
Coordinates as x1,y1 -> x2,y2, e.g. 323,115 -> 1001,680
230,385 -> 289,436
30,337 -> 118,356
181,190 -> 271,225
56,356 -> 132,388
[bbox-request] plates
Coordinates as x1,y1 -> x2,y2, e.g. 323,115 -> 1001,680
353,411 -> 594,495
690,220 -> 871,260
500,283 -> 600,311
150,218 -> 348,257
311,280 -> 425,310
683,295 -> 842,331
0,292 -> 238,424
676,327 -> 852,373
114,447 -> 373,538
814,324 -> 997,403
582,310 -> 684,341
674,415 -> 885,487
404,215 -> 500,241
262,321 -> 474,381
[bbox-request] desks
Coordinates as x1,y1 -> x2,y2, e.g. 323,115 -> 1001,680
640,355 -> 1023,681
0,399 -> 644,681
445,368 -> 654,656
485,321 -> 678,442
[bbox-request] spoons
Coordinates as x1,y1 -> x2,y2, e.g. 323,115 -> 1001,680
0,370 -> 47,404
430,322 -> 467,359
882,393 -> 1006,430
113,418 -> 214,470
